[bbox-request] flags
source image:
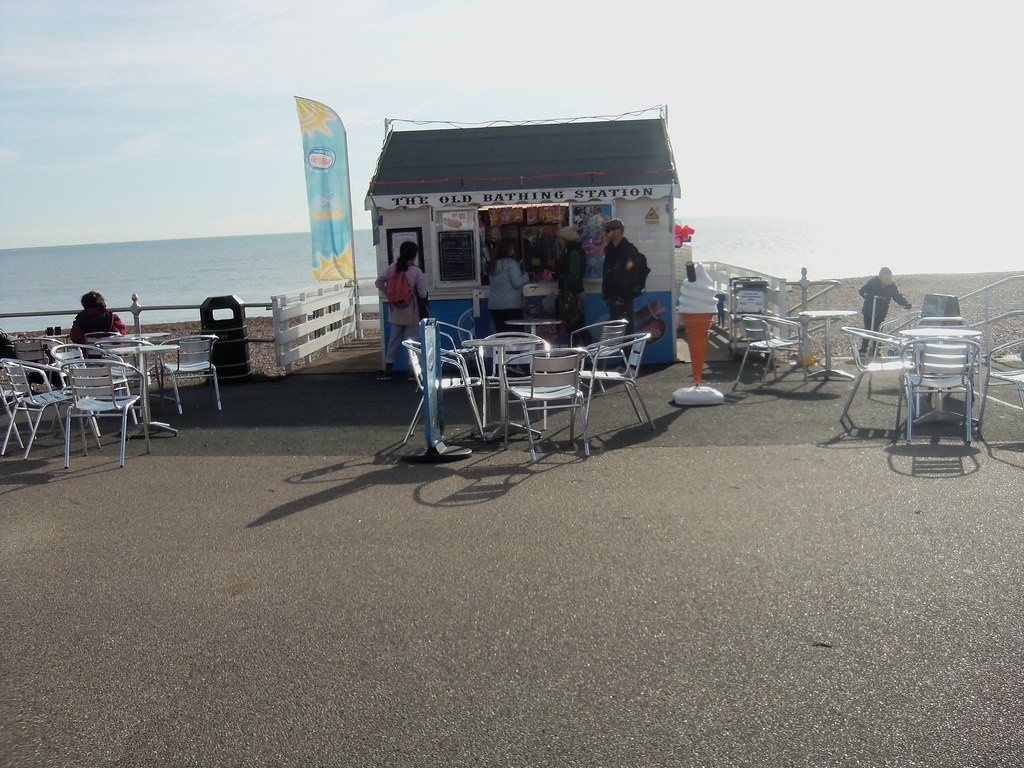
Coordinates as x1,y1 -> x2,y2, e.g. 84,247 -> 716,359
294,96 -> 357,282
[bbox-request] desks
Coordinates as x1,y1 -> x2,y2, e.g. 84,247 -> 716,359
104,346 -> 181,438
798,311 -> 860,383
109,332 -> 177,417
462,337 -> 544,440
506,320 -> 562,375
900,328 -> 982,426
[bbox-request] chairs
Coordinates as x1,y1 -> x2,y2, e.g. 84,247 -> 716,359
0,329 -> 222,469
394,319 -> 654,460
731,295 -> 1024,441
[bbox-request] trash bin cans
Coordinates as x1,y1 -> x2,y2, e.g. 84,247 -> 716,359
200,294 -> 252,386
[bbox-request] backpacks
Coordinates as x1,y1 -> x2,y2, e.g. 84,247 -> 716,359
384,262 -> 413,308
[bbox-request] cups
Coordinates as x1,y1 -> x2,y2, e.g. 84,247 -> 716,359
55,327 -> 61,335
45,327 -> 54,336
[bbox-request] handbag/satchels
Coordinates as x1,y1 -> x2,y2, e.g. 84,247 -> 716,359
556,289 -> 586,320
619,277 -> 644,297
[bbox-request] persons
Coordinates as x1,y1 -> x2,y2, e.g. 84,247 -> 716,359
602,219 -> 642,378
375,242 -> 428,381
858,267 -> 912,357
555,226 -> 593,366
487,237 -> 530,375
70,291 -> 126,359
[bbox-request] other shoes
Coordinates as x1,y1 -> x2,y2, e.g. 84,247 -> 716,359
376,375 -> 393,380
506,364 -> 527,375
408,374 -> 422,382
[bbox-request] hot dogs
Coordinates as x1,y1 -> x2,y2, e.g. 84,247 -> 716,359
443,218 -> 463,229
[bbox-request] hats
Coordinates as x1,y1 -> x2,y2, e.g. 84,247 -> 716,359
603,220 -> 623,230
558,226 -> 579,242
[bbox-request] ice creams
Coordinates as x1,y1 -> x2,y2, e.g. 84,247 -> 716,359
678,261 -> 719,385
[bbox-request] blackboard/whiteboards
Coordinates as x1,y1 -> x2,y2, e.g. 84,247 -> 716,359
921,294 -> 947,327
439,230 -> 478,281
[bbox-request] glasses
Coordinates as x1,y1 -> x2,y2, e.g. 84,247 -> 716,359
605,228 -> 617,233
